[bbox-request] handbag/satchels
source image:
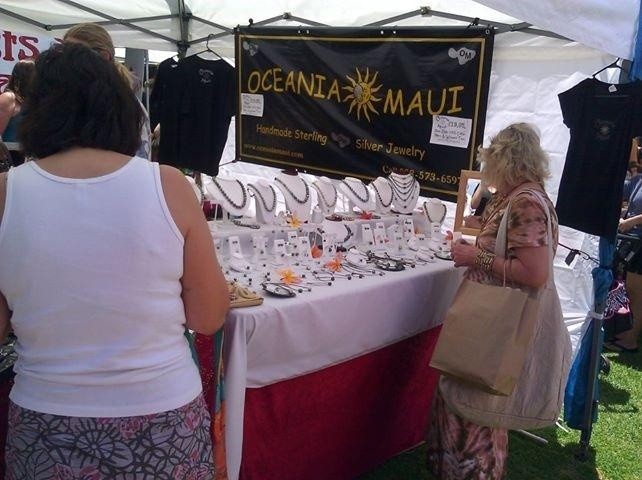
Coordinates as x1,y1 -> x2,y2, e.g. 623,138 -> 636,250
439,271 -> 572,429
429,278 -> 540,395
615,233 -> 639,264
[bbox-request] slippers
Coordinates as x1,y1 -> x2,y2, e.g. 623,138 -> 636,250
605,334 -> 619,341
603,342 -> 638,353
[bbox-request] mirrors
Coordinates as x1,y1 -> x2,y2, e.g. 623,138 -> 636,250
453,169 -> 505,235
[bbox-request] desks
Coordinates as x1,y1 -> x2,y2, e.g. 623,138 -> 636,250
189,222 -> 532,480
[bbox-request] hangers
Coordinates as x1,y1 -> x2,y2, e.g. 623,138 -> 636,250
161,34 -> 231,68
561,54 -> 641,103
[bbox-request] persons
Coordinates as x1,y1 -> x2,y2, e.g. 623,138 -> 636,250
425,122 -> 559,480
469,165 -> 488,217
624,161 -> 640,180
1,21 -> 162,177
1,42 -> 231,480
602,171 -> 642,357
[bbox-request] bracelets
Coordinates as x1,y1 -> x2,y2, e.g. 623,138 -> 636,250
474,249 -> 495,275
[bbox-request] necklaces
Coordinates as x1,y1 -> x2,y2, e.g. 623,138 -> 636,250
370,182 -> 394,207
210,173 -> 248,212
423,200 -> 447,225
340,178 -> 371,204
273,175 -> 310,204
247,183 -> 278,213
312,179 -> 338,208
316,223 -> 353,244
388,172 -> 418,209
